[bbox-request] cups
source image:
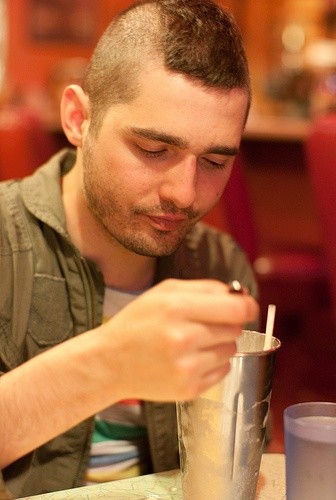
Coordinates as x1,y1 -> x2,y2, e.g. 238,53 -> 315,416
177,330 -> 281,500
283,403 -> 336,500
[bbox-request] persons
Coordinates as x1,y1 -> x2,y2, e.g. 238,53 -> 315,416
0,0 -> 274,500
